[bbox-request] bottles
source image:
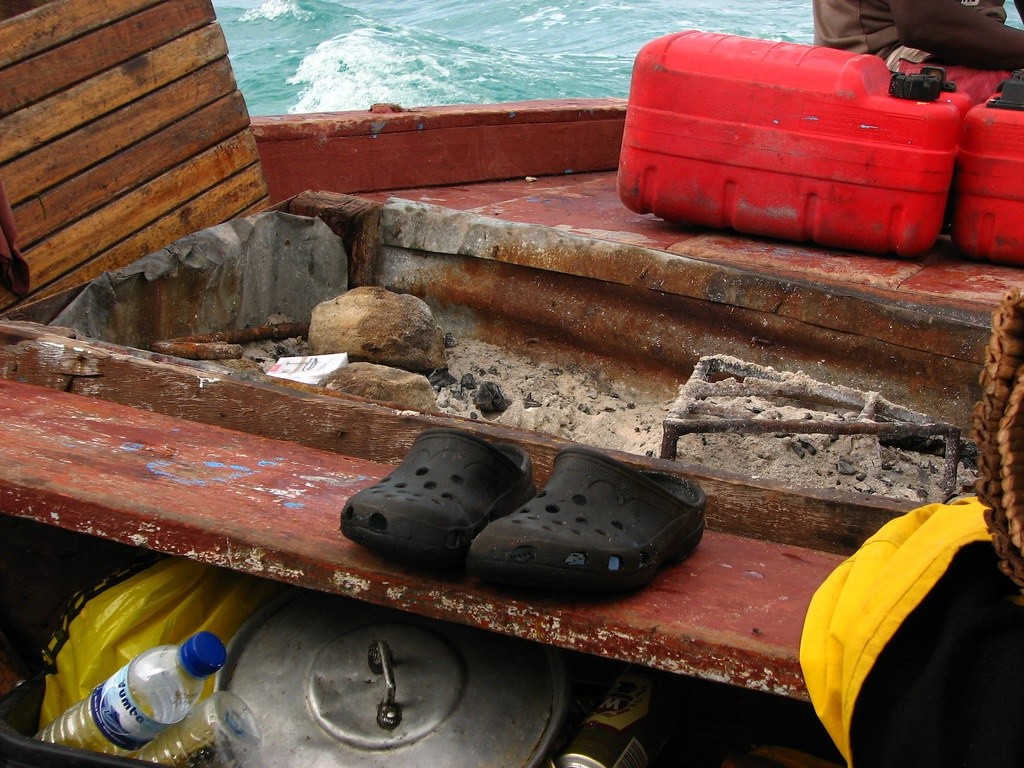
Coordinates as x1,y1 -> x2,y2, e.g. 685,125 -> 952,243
125,690 -> 262,768
953,67 -> 1024,267
29,630 -> 227,759
614,29 -> 972,257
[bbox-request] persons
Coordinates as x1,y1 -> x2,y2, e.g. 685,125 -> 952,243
812,0 -> 1024,103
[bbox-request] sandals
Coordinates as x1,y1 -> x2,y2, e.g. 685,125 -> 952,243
339,427 -> 705,593
